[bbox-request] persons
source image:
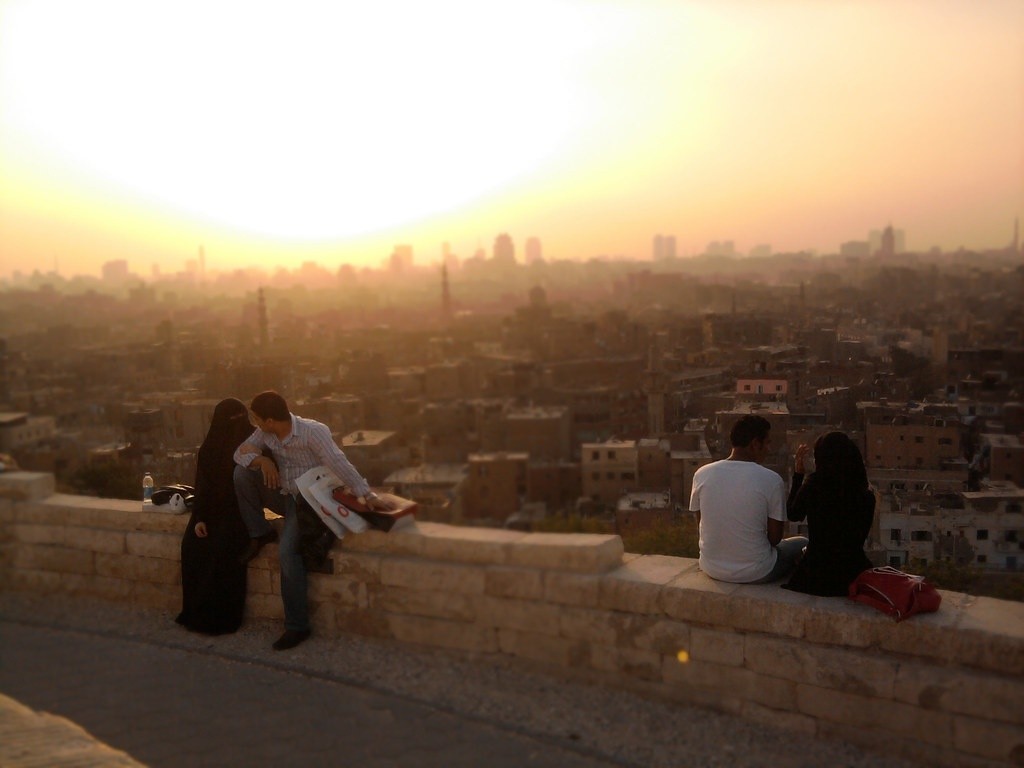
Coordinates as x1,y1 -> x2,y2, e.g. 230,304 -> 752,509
689,415 -> 809,583
236,392 -> 394,650
787,433 -> 875,596
172,398 -> 272,636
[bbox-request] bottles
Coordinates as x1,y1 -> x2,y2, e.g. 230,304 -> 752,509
143,472 -> 153,504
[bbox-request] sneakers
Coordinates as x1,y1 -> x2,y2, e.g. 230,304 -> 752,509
273,629 -> 312,650
243,530 -> 277,561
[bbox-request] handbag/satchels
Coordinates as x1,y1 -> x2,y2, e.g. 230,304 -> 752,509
293,467 -> 417,539
850,566 -> 941,622
153,483 -> 194,515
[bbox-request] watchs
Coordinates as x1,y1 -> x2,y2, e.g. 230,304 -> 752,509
366,492 -> 378,501
